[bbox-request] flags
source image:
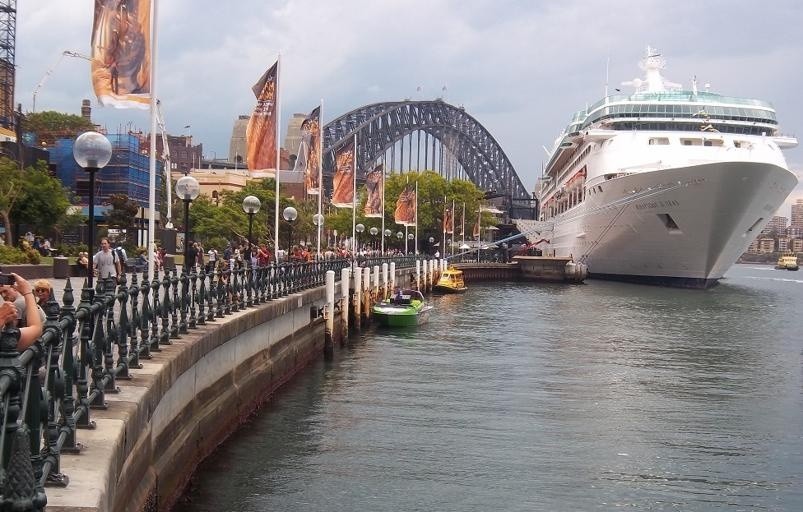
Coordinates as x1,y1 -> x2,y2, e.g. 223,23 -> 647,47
300,106 -> 322,194
365,163 -> 384,218
331,140 -> 356,208
394,186 -> 417,227
246,53 -> 279,178
90,0 -> 158,110
441,204 -> 480,240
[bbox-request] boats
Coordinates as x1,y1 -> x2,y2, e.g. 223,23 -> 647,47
371,288 -> 432,327
774,251 -> 799,271
434,264 -> 470,293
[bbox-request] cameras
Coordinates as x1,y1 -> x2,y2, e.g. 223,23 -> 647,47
0,274 -> 16,285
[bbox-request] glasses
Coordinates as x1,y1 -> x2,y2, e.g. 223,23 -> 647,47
36,288 -> 44,293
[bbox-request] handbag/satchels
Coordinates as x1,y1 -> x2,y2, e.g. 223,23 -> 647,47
112,250 -> 127,285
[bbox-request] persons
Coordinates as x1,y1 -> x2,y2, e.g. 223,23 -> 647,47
689,75 -> 698,102
282,241 -> 413,265
104,0 -> 146,95
693,106 -> 718,131
89,238 -> 121,317
114,241 -> 127,272
0,272 -> 54,480
435,250 -> 449,258
181,237 -> 270,280
472,238 -> 535,262
77,252 -> 97,276
19,232 -> 50,256
143,243 -> 166,272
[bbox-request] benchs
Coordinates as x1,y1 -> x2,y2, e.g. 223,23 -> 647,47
135,257 -> 165,270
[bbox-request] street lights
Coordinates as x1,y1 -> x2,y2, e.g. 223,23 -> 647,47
370,227 -> 378,265
283,206 -> 297,287
429,236 -> 487,265
242,195 -> 262,290
313,214 -> 324,275
408,234 -> 414,266
175,175 -> 200,315
73,131 -> 113,369
397,231 -> 403,268
356,223 -> 365,267
384,229 -> 391,263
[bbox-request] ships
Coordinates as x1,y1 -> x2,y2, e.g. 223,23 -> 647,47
530,42 -> 799,291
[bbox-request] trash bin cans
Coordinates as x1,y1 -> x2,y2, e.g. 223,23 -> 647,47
53,256 -> 69,279
163,255 -> 175,271
